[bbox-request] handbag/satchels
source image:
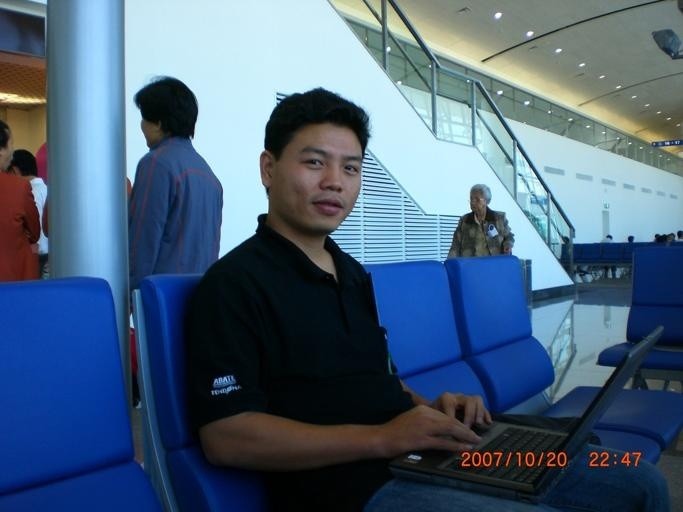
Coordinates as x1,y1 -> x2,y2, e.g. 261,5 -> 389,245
457,406 -> 602,445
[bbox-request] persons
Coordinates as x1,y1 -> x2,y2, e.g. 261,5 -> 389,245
175,85 -> 493,512
1,120 -> 42,284
600,234 -> 617,279
4,148 -> 48,275
124,74 -> 223,412
653,230 -> 683,243
627,235 -> 634,242
443,182 -> 516,262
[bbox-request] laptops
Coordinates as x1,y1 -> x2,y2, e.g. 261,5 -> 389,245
389,324 -> 666,504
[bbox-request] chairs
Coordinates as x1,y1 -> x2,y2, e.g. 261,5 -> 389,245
132,270 -> 253,512
1,275 -> 160,511
443,254 -> 682,446
595,245 -> 682,391
367,260 -> 662,465
